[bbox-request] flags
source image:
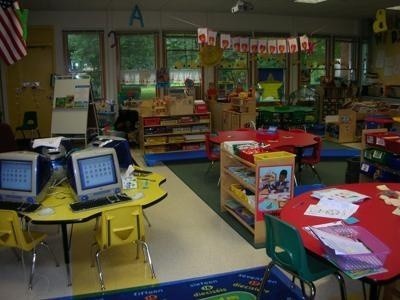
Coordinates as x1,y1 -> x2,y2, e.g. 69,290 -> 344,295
1,1 -> 29,66
197,28 -> 310,54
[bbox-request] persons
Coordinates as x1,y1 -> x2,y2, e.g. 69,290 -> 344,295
268,170 -> 290,193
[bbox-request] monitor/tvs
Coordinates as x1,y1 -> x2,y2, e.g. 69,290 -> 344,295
0,151 -> 50,204
68,148 -> 122,202
88,136 -> 133,168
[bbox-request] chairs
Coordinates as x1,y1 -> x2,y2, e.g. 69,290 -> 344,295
114,110 -> 138,147
205,128 -> 323,187
100,139 -> 152,227
90,204 -> 157,291
257,214 -> 347,300
0,210 -> 60,291
16,111 -> 41,139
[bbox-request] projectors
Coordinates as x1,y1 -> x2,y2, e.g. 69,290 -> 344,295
231,4 -> 254,14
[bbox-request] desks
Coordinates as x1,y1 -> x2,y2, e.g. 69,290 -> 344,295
256,106 -> 315,130
18,169 -> 168,287
280,182 -> 400,300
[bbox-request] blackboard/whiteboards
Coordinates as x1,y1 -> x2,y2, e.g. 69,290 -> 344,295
50,79 -> 91,134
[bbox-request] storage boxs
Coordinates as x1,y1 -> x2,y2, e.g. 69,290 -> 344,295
165,95 -> 194,115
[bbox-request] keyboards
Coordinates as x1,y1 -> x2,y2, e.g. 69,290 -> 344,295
0,201 -> 40,212
70,193 -> 131,213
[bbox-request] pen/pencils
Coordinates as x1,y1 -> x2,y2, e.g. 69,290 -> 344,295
292,201 -> 305,209
137,178 -> 156,181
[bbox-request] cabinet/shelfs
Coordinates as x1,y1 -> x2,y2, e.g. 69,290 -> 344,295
220,142 -> 295,244
315,85 -> 400,183
139,112 -> 211,166
222,111 -> 256,131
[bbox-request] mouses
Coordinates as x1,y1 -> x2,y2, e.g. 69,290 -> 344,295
38,208 -> 55,215
132,193 -> 143,201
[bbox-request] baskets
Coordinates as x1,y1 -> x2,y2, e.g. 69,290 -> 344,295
318,226 -> 389,271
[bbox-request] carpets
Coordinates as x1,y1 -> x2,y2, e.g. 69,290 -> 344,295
161,141 -> 381,249
70,218 -> 158,297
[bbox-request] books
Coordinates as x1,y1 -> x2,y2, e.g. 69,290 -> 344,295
326,256 -> 389,280
